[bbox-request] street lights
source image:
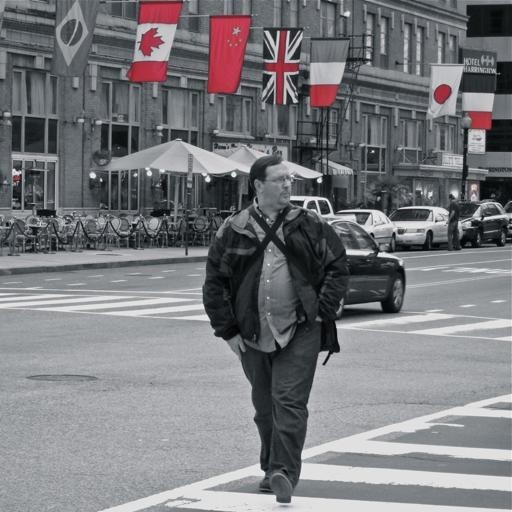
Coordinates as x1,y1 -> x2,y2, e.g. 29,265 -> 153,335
459,113 -> 472,200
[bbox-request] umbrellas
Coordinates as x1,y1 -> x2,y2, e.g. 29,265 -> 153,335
226,146 -> 323,181
89,138 -> 250,234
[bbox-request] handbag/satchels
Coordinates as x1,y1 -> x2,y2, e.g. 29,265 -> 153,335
320,319 -> 342,355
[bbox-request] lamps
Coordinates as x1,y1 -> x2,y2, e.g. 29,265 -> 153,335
0,107 -> 14,129
144,122 -> 165,139
72,109 -> 106,131
345,140 -> 368,154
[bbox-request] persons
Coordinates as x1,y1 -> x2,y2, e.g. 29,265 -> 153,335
202,154 -> 350,504
446,192 -> 463,251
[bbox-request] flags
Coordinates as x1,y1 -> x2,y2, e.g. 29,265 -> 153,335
461,73 -> 497,129
54,0 -> 99,76
310,38 -> 350,107
125,0 -> 182,82
262,26 -> 304,105
208,14 -> 251,94
426,64 -> 464,119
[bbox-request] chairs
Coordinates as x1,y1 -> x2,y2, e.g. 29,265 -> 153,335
0,201 -> 237,256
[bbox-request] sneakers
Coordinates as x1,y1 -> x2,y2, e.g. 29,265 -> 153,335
269,472 -> 294,504
258,476 -> 274,493
446,246 -> 462,251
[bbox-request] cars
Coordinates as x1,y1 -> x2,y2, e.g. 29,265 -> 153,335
289,195 -> 512,255
311,215 -> 409,316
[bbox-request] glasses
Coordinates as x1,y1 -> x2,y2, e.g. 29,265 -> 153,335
262,174 -> 292,184
